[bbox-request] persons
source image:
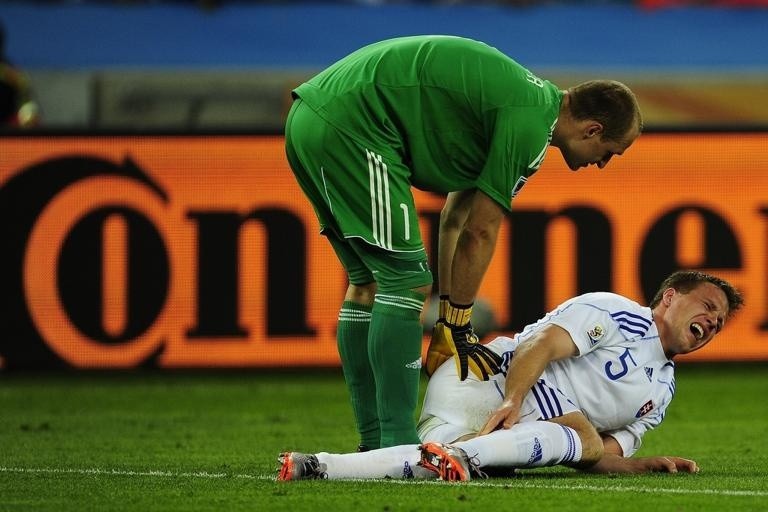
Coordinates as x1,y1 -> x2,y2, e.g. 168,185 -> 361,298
283,33 -> 647,455
274,266 -> 746,484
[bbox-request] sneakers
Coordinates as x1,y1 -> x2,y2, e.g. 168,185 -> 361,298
274,451 -> 329,483
417,442 -> 489,482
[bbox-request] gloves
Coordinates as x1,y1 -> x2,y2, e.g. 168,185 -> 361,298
426,295 -> 454,377
444,300 -> 503,381
